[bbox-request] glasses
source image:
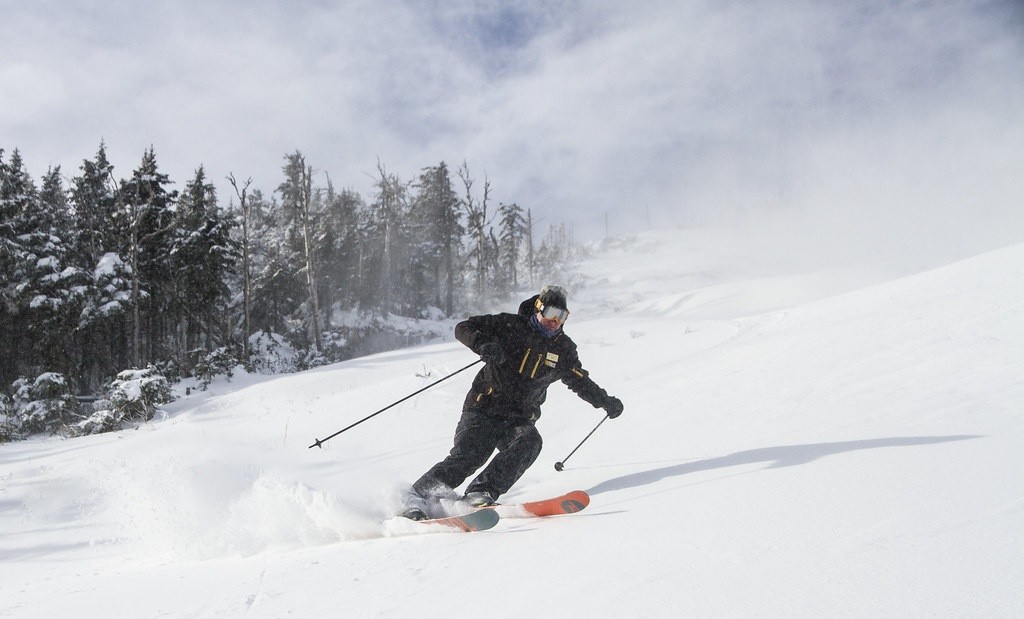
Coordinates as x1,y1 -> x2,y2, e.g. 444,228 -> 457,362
540,304 -> 569,324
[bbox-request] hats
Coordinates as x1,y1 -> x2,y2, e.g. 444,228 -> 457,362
538,284 -> 567,309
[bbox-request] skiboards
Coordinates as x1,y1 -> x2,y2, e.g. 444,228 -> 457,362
381,490 -> 591,532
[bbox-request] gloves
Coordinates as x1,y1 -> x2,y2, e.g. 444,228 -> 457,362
476,336 -> 506,369
601,394 -> 624,419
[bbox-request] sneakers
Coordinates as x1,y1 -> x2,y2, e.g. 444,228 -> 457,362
403,487 -> 427,521
461,491 -> 494,508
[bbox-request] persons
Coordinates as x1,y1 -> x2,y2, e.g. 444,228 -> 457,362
400,286 -> 623,520
